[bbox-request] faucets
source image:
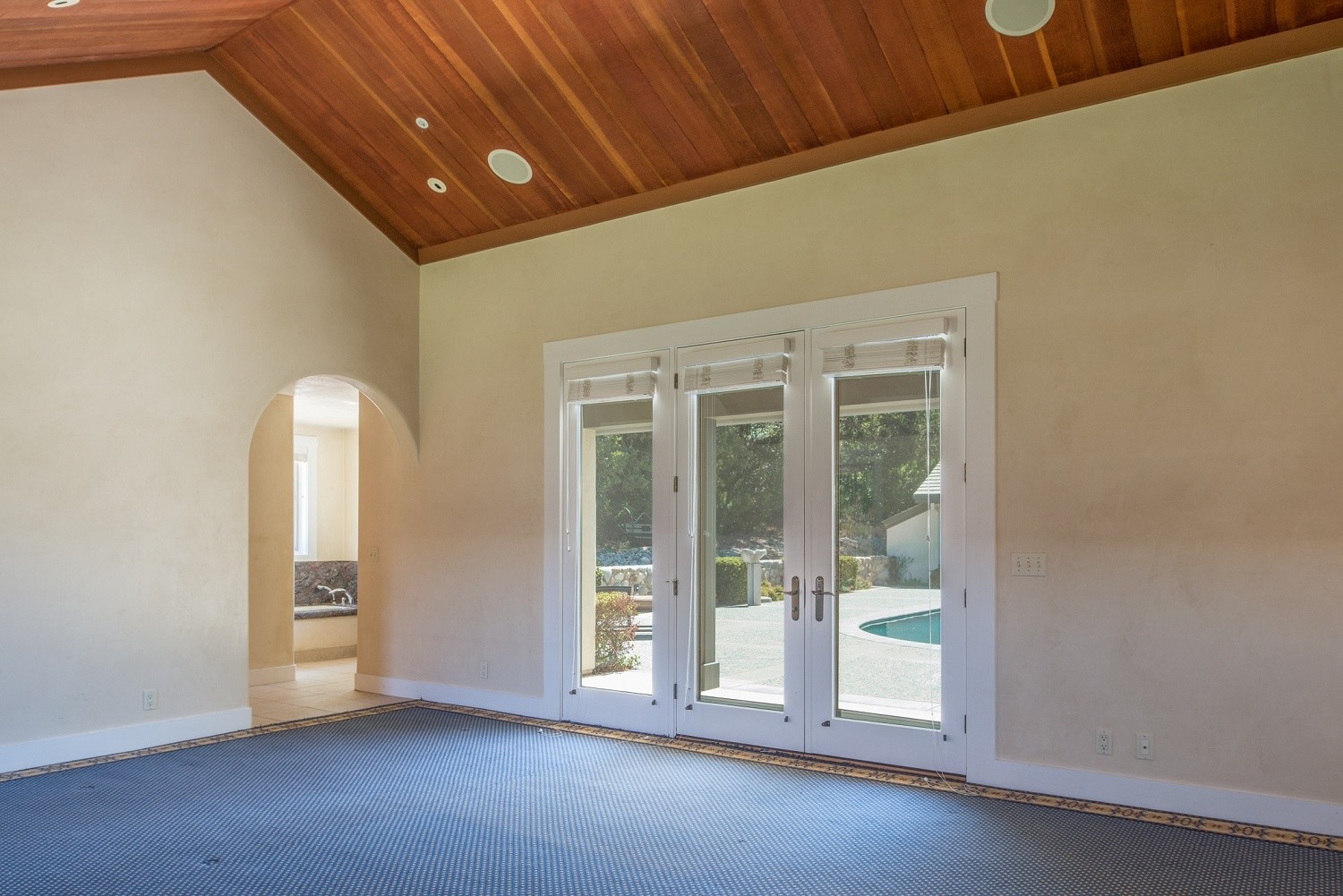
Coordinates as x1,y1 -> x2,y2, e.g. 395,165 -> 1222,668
329,587 -> 354,604
313,584 -> 336,604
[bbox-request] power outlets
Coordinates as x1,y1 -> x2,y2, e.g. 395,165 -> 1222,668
1136,733 -> 1153,760
1011,553 -> 1046,576
368,545 -> 379,562
1096,730 -> 1112,755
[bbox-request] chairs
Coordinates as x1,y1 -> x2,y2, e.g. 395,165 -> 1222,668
596,586 -> 651,636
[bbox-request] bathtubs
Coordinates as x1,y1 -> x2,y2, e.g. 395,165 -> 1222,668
294,603 -> 358,620
